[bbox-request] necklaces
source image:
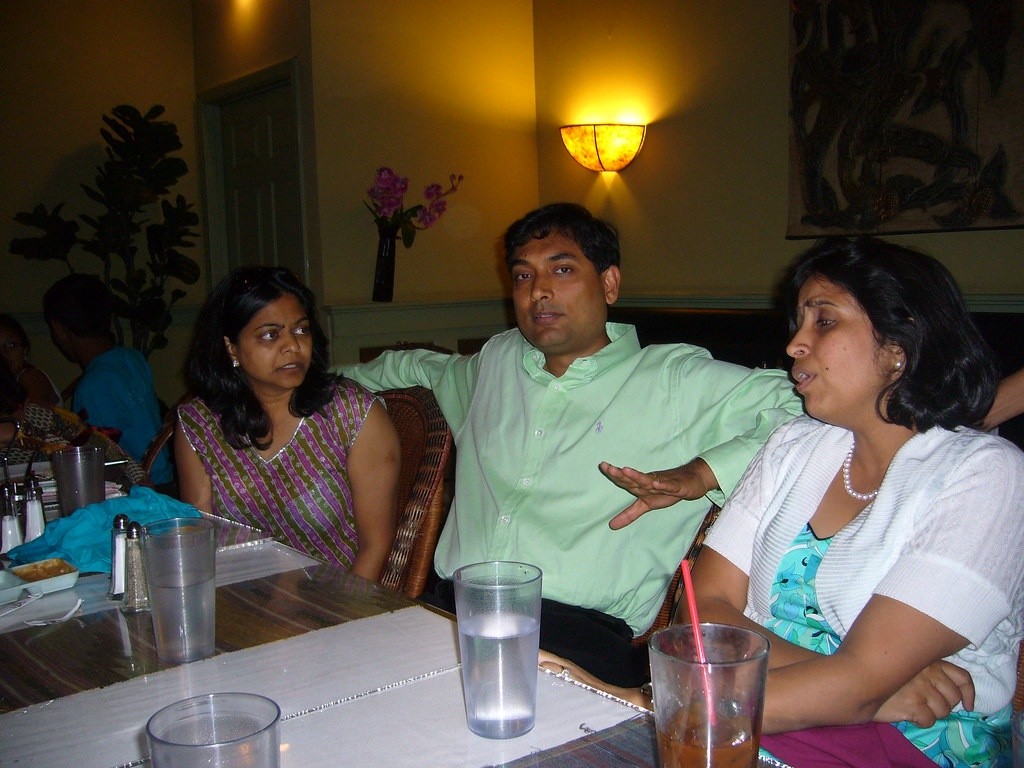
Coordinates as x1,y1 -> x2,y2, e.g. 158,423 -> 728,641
844,452 -> 880,501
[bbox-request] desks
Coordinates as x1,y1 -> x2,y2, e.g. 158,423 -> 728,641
1,511 -> 795,768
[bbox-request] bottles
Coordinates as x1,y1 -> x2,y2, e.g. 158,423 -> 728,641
105,513 -> 130,601
119,520 -> 152,614
24,477 -> 46,543
0,485 -> 23,555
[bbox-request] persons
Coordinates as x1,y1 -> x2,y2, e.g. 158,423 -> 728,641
326,202 -> 805,687
669,232 -> 1023,768
0,313 -> 66,444
43,272 -> 174,498
174,266 -> 402,586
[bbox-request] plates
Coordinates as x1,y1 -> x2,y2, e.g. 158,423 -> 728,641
0,558 -> 80,606
0,461 -> 57,488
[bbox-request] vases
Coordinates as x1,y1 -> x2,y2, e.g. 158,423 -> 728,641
372,222 -> 402,302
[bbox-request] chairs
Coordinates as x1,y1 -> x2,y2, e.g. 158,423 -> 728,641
142,391 -> 194,495
375,386 -> 453,601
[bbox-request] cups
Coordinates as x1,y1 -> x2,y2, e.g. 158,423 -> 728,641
146,691 -> 283,768
451,560 -> 543,740
52,445 -> 107,518
649,620 -> 771,768
137,516 -> 217,664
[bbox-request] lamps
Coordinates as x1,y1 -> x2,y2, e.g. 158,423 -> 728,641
558,123 -> 647,173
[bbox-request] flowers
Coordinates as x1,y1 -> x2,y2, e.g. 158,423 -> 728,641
361,166 -> 463,250
